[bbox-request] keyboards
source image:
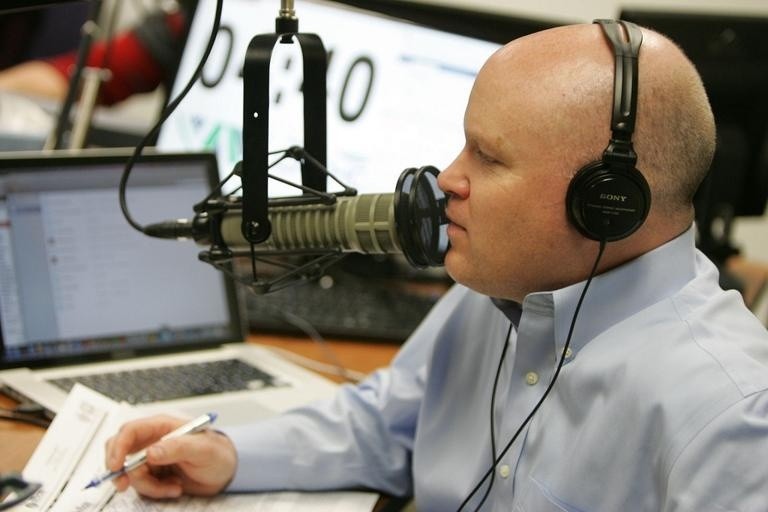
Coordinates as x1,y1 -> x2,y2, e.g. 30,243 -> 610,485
235,265 -> 441,341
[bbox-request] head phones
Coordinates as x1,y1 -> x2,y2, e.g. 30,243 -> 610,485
567,18 -> 652,243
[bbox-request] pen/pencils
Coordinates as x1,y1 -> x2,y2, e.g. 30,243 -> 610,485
84,413 -> 215,488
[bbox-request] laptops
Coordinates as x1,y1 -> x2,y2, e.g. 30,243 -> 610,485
0,146 -> 345,431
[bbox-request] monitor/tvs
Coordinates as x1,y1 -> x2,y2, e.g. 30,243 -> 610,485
154,1 -> 506,270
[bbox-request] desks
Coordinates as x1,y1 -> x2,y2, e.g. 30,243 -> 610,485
0,332 -> 413,512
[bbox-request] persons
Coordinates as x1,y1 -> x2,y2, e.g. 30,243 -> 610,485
97,17 -> 768,512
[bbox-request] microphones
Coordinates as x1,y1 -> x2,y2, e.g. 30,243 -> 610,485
192,166 -> 445,270
